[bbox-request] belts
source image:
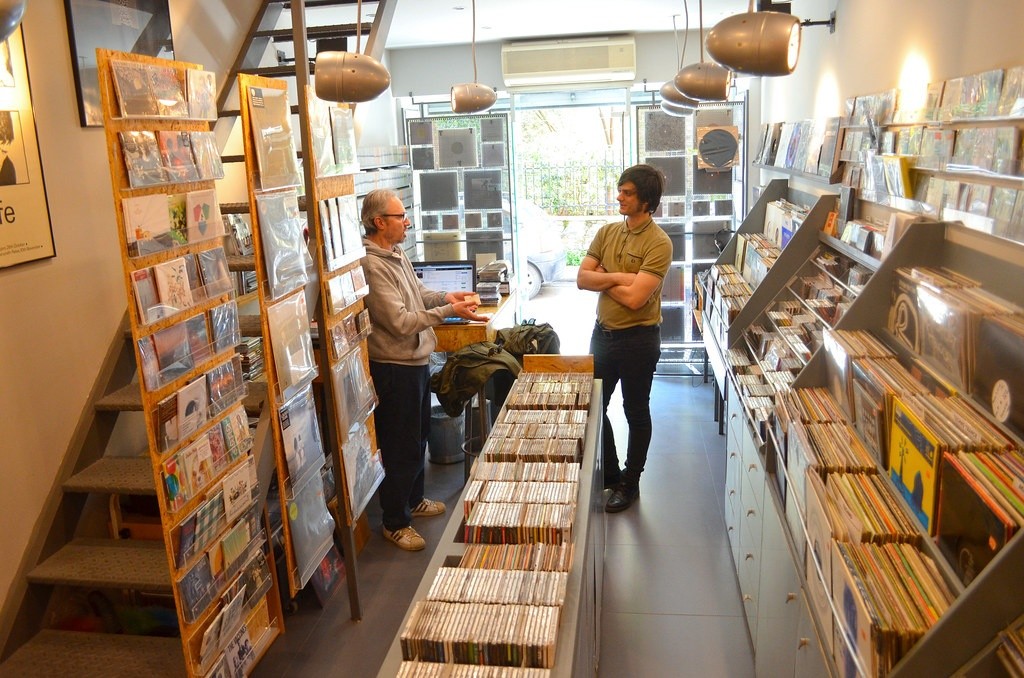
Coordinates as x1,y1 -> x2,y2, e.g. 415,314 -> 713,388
594,320 -> 660,339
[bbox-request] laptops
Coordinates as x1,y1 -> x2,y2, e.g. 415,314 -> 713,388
409,260 -> 476,324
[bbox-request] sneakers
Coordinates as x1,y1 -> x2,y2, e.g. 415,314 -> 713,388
382,524 -> 426,551
410,498 -> 447,517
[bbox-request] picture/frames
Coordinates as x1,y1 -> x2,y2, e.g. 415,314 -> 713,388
0,20 -> 57,270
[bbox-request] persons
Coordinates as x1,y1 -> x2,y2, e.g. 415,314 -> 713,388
577,165 -> 673,514
361,189 -> 489,551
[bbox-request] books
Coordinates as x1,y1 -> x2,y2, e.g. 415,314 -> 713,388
703,68 -> 1024,678
108,57 -> 273,678
396,370 -> 592,678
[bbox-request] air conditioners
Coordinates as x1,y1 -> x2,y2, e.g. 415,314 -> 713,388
501,35 -> 637,88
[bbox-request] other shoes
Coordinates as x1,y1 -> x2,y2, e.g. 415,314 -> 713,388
605,480 -> 640,513
604,466 -> 621,488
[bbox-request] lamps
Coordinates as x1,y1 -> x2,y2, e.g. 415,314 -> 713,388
450,0 -> 496,113
659,0 -> 801,117
314,0 -> 391,102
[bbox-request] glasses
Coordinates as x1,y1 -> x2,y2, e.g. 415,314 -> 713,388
382,212 -> 409,221
468,341 -> 502,357
521,318 -> 536,326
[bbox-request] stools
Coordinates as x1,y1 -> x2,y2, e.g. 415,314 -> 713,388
431,319 -> 561,486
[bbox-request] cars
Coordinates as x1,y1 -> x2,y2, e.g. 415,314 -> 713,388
457,192 -> 568,301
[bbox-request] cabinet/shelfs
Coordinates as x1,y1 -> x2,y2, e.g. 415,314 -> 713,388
693,117 -> 1024,678
